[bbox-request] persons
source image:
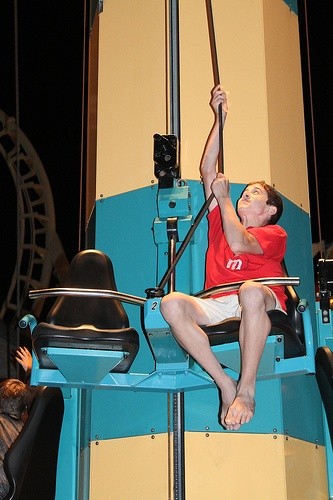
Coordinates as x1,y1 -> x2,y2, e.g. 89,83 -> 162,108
0,347 -> 33,500
159,85 -> 287,430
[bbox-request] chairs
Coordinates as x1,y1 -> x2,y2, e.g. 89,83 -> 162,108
197,255 -> 299,359
36,249 -> 141,375
